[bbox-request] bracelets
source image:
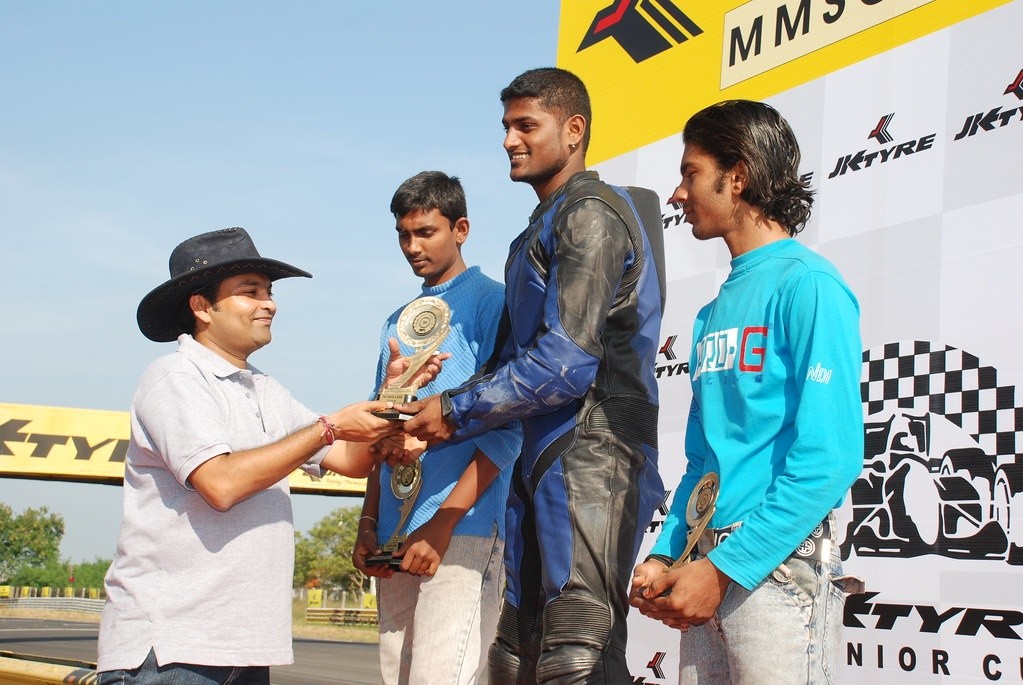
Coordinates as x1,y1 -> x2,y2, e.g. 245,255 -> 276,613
360,516 -> 378,526
314,416 -> 341,445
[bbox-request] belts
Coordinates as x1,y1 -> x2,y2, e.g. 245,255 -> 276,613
690,511 -> 830,562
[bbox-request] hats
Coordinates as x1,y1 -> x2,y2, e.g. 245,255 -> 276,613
137,227 -> 313,342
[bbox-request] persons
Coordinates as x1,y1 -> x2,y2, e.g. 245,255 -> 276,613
97,226 -> 452,685
353,169 -> 523,685
368,68 -> 664,685
628,99 -> 865,685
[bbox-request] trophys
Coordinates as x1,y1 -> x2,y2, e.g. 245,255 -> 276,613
364,459 -> 423,572
639,472 -> 720,597
371,296 -> 450,420
